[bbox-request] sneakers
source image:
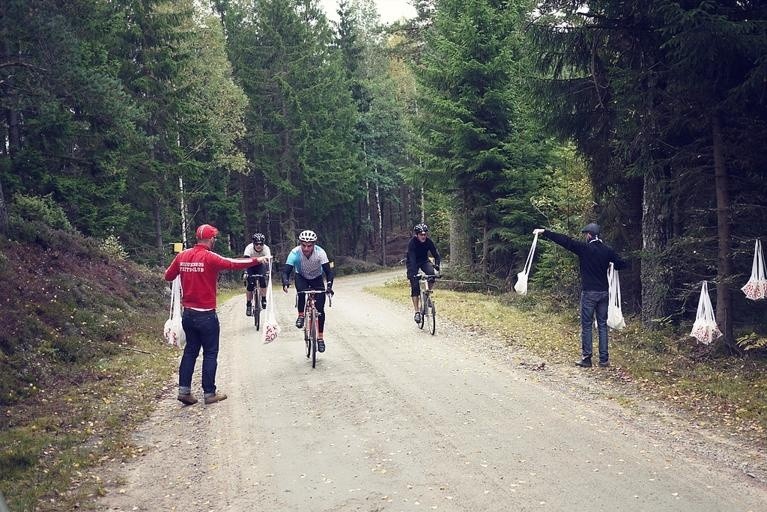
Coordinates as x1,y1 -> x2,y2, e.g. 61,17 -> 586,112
262,297 -> 266,308
317,337 -> 325,352
575,359 -> 592,367
205,393 -> 227,404
600,360 -> 610,366
178,394 -> 197,404
415,312 -> 420,323
247,305 -> 252,316
296,316 -> 304,328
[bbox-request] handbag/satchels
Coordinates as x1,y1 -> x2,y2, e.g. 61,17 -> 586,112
594,303 -> 626,329
741,279 -> 767,301
262,320 -> 281,344
164,317 -> 186,350
690,322 -> 723,345
513,271 -> 528,296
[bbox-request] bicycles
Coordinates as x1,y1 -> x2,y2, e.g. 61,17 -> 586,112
241,271 -> 270,330
408,274 -> 442,335
295,291 -> 332,367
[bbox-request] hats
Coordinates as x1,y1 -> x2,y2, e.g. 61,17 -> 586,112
582,224 -> 599,234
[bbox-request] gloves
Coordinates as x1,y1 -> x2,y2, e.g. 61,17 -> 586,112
433,265 -> 439,271
413,272 -> 421,280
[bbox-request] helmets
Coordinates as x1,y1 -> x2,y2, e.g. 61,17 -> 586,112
252,233 -> 265,244
196,224 -> 218,239
414,224 -> 428,235
299,230 -> 317,242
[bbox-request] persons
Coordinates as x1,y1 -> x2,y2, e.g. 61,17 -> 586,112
279,227 -> 335,352
161,222 -> 274,404
532,221 -> 627,370
406,222 -> 441,323
239,230 -> 273,317
